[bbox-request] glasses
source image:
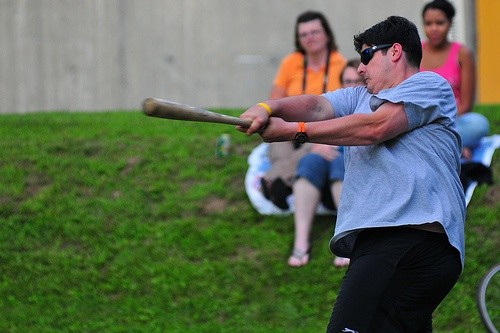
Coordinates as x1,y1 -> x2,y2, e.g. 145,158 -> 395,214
360,43 -> 410,66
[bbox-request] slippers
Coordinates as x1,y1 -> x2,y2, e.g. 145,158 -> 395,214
288,241 -> 312,266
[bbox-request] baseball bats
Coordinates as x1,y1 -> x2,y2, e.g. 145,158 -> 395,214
142,97 -> 267,131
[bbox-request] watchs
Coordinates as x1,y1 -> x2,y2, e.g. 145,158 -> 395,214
295,119 -> 308,144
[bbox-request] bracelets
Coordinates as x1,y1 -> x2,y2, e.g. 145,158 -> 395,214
256,102 -> 272,116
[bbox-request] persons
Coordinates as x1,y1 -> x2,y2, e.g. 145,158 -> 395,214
411,0 -> 490,173
286,59 -> 368,268
264,8 -> 352,208
236,16 -> 466,333
